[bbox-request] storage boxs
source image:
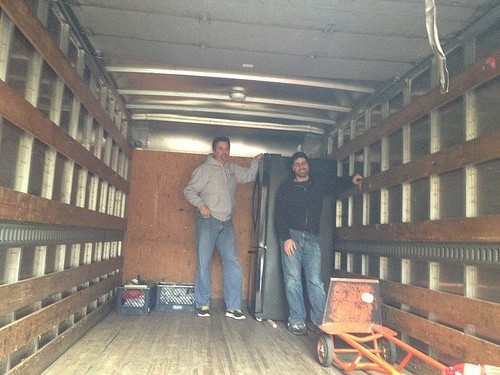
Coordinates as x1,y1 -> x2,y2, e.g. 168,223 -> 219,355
155,281 -> 196,312
115,282 -> 155,315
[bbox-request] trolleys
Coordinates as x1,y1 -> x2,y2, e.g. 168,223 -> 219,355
315,277 -> 448,375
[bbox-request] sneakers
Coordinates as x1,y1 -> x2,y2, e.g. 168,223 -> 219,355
225,309 -> 246,320
197,306 -> 211,316
288,321 -> 307,334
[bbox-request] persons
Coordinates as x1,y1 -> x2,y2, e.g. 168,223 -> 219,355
183,137 -> 264,320
275,152 -> 364,334
